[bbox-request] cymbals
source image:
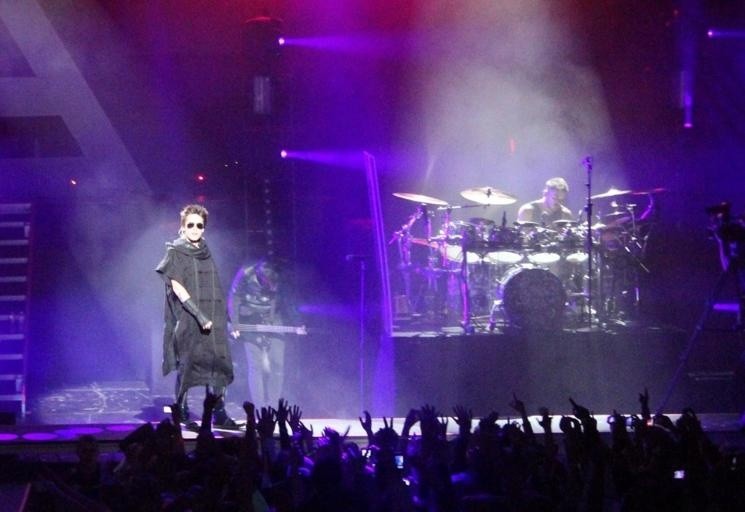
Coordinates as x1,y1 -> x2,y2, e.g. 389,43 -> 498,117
462,187 -> 516,205
631,187 -> 665,196
590,189 -> 633,199
394,193 -> 448,207
602,217 -> 629,241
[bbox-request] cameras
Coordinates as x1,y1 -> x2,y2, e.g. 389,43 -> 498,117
626,418 -> 635,426
673,469 -> 684,478
394,453 -> 404,469
607,417 -> 614,422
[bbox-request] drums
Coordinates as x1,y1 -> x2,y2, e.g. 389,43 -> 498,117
487,225 -> 524,265
563,226 -> 590,264
526,227 -> 562,264
498,264 -> 566,328
439,221 -> 481,264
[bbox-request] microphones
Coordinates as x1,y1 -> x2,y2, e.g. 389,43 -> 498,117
583,155 -> 593,164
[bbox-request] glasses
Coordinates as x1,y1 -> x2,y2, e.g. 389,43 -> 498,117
184,223 -> 205,229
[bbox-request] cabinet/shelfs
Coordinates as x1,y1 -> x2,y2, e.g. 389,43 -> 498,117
0,193 -> 39,422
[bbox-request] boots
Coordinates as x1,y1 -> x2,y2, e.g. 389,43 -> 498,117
177,402 -> 191,421
213,409 -> 243,429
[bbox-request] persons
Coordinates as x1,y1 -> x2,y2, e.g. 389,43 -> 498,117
155,204 -> 244,431
1,386 -> 744,512
708,219 -> 745,341
515,174 -> 576,240
225,257 -> 308,417
